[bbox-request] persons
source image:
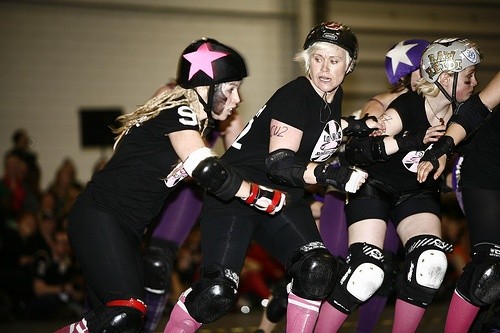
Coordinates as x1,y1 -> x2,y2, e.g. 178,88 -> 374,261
0,20 -> 500,333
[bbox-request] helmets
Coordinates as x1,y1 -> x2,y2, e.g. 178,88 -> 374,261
303,21 -> 358,60
177,38 -> 248,89
420,38 -> 483,83
385,40 -> 429,84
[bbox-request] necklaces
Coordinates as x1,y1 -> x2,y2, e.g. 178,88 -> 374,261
426,98 -> 450,125
